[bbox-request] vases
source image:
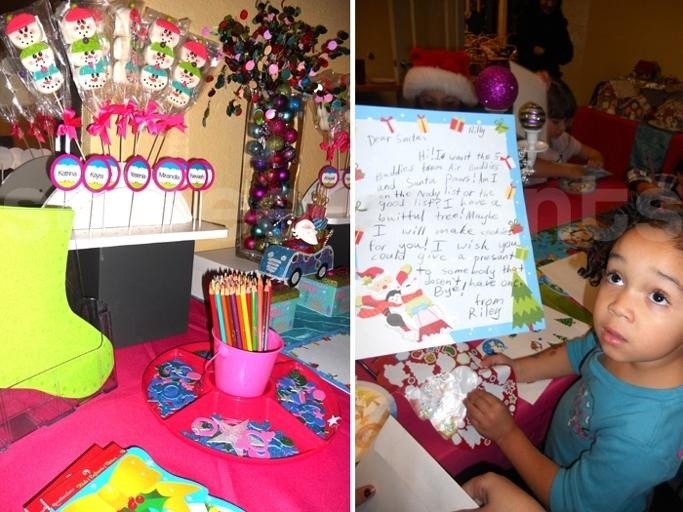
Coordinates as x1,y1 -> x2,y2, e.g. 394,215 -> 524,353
235,96 -> 307,262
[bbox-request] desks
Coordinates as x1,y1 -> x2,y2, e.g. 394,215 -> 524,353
356,175 -> 683,511
0,245 -> 352,512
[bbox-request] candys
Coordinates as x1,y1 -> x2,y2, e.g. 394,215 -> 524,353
0,0 -> 350,251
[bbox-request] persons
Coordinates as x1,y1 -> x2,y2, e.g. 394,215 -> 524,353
355,471 -> 545,512
532,79 -> 604,178
464,213 -> 683,512
625,159 -> 683,215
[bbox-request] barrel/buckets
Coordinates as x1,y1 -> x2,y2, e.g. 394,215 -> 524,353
206,322 -> 284,396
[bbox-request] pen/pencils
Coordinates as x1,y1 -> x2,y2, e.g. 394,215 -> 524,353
208,266 -> 273,353
